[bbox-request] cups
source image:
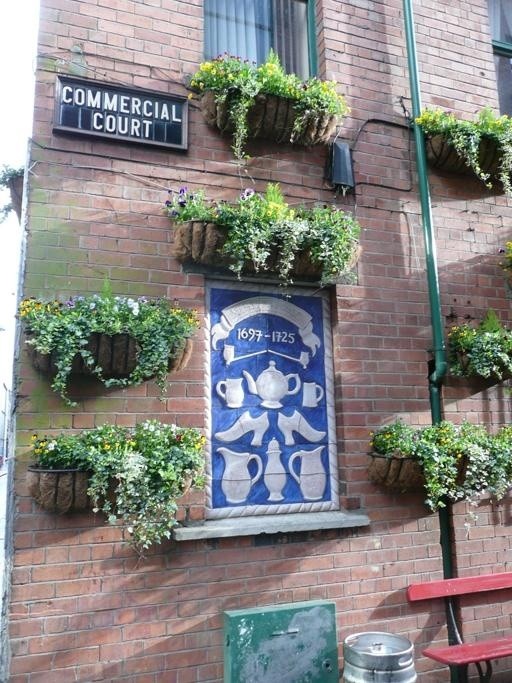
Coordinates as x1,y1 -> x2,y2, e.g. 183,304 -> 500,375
216,379 -> 243,409
302,383 -> 323,407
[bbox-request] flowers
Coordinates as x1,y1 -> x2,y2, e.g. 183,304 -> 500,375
494,239 -> 512,297
367,418 -> 512,538
445,306 -> 512,385
188,51 -> 354,173
14,294 -> 202,399
162,182 -> 363,302
27,416 -> 210,565
414,105 -> 512,206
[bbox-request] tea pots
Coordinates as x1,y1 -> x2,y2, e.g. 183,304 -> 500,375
242,360 -> 301,409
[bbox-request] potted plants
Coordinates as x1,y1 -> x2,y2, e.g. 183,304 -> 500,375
0,162 -> 24,224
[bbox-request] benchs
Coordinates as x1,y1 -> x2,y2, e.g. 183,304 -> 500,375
406,571 -> 512,683
